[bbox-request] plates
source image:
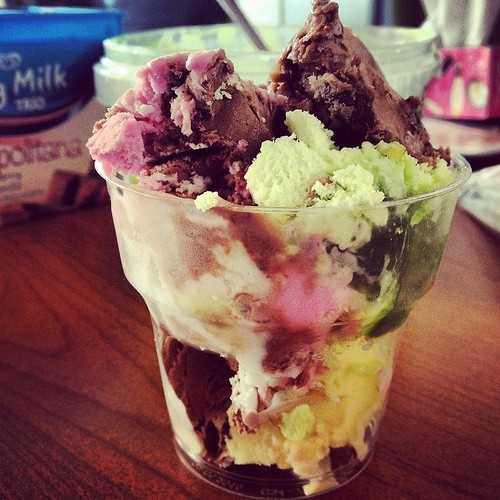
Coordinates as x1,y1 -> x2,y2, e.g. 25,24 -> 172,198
458,166 -> 500,232
420,117 -> 500,156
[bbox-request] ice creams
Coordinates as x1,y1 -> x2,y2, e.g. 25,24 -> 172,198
85,0 -> 460,500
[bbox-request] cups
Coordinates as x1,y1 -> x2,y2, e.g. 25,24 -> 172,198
92,18 -> 442,109
93,139 -> 472,499
